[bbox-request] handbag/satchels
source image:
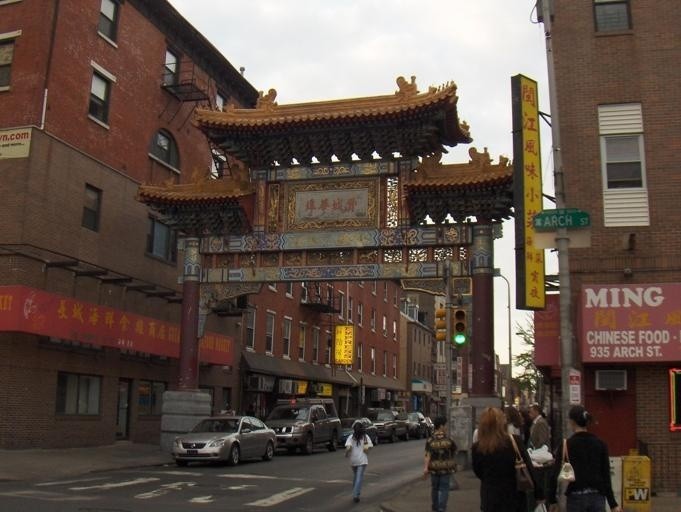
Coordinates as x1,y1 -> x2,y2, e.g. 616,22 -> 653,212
544,438 -> 575,504
363,436 -> 369,453
508,432 -> 535,491
449,475 -> 459,490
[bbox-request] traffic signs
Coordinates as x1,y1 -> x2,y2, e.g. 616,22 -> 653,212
533,207 -> 591,230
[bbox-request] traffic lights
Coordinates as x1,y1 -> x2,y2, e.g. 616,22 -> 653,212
451,306 -> 468,348
434,307 -> 447,341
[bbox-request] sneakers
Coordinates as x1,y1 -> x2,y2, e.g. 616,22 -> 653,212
353,495 -> 360,502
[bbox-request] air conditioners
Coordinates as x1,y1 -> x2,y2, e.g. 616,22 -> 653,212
595,369 -> 627,391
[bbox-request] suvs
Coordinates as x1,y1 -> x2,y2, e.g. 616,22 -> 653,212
265,398 -> 343,453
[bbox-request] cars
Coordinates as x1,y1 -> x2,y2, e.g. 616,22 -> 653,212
172,415 -> 278,465
341,406 -> 436,445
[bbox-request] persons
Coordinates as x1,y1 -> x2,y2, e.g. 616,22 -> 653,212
421,415 -> 457,512
343,421 -> 373,503
469,400 -> 561,512
544,405 -> 625,512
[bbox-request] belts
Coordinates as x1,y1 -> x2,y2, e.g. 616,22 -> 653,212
571,486 -> 600,495
